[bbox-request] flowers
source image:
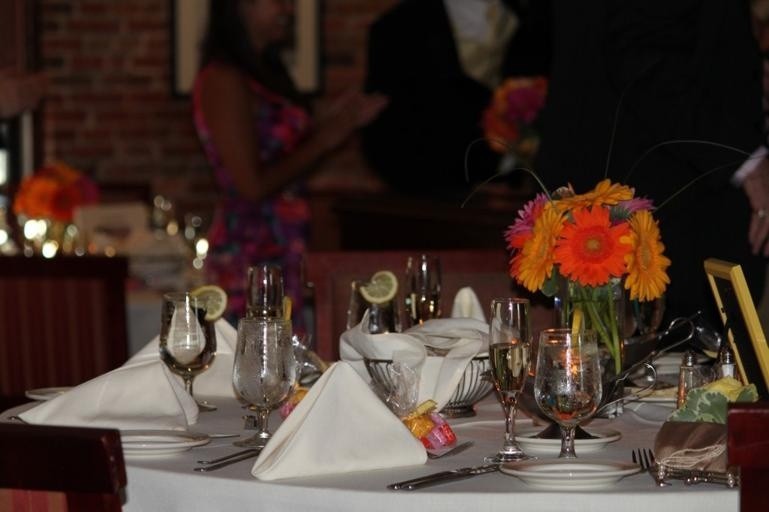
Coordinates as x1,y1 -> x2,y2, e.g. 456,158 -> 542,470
482,74 -> 552,165
9,162 -> 99,221
502,176 -> 671,366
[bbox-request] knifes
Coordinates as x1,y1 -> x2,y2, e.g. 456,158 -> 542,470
388,463 -> 500,492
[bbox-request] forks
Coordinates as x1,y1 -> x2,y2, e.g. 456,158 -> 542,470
631,448 -> 673,487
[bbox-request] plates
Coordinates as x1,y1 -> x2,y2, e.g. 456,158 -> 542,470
654,351 -> 712,375
625,393 -> 679,428
118,427 -> 211,457
514,424 -> 622,455
499,455 -> 642,489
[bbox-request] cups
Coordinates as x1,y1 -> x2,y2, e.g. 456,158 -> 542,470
345,280 -> 401,334
246,264 -> 286,320
677,366 -> 703,409
402,255 -> 444,326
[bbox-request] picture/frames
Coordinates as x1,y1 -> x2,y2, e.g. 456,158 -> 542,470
168,0 -> 328,100
702,256 -> 767,400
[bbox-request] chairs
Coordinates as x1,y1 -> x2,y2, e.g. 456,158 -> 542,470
309,248 -> 514,359
725,401 -> 769,511
1,252 -> 133,399
2,421 -> 131,512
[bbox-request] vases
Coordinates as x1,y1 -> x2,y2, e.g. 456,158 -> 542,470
563,274 -> 629,415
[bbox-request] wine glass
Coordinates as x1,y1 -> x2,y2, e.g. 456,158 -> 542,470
490,297 -> 533,463
231,317 -> 297,450
533,329 -> 603,459
159,293 -> 217,413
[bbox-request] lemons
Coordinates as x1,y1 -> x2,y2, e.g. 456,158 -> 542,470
190,285 -> 228,321
360,270 -> 398,304
283,297 -> 292,333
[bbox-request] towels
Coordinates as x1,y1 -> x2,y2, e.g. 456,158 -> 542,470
122,315 -> 243,398
452,286 -> 486,323
340,312 -> 481,414
16,361 -> 202,434
251,361 -> 429,481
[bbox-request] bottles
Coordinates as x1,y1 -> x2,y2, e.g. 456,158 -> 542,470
719,351 -> 736,381
683,350 -> 702,389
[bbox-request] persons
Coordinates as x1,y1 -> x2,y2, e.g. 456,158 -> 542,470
1,67 -> 53,120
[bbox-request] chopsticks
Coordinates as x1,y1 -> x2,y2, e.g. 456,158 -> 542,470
26,387 -> 70,402
